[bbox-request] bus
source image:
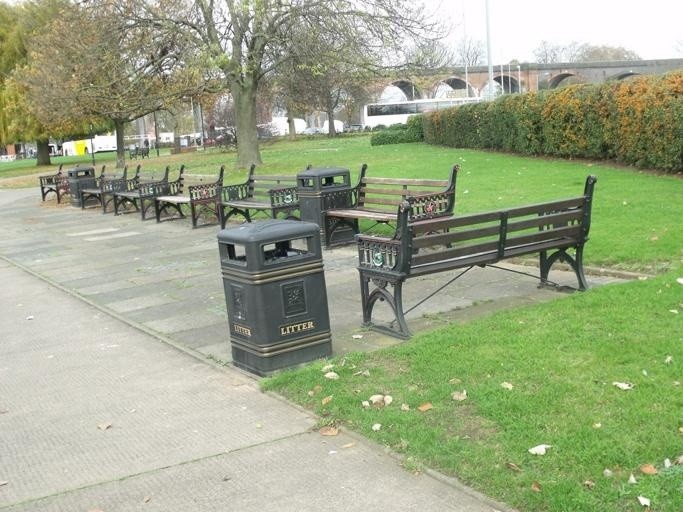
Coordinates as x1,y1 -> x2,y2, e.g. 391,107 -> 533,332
363,97 -> 480,127
61,128 -> 117,156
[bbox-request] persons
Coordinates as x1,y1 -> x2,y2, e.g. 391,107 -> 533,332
28,148 -> 33,159
144,139 -> 149,148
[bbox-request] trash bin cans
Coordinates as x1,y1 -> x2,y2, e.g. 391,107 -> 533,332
217,219 -> 334,378
296,167 -> 355,249
67,167 -> 97,208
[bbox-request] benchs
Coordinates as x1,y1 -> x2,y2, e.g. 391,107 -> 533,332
38,162 -> 172,223
215,164 -> 314,233
151,163 -> 226,230
357,173 -> 600,340
321,163 -> 467,255
